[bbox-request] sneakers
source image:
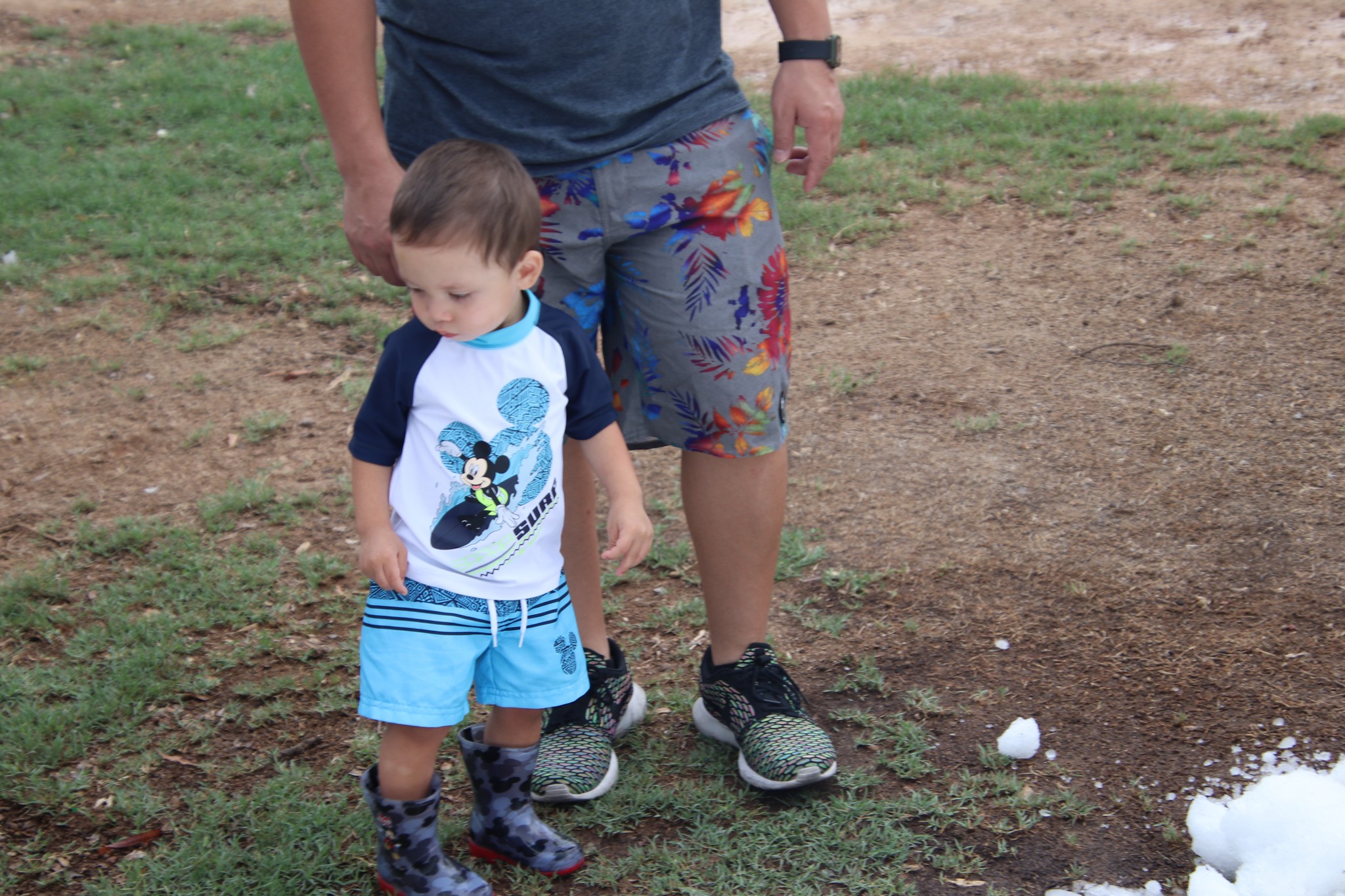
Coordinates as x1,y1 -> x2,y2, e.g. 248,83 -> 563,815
530,638 -> 648,802
692,642 -> 833,790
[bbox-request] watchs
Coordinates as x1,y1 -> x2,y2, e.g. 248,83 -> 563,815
778,35 -> 842,69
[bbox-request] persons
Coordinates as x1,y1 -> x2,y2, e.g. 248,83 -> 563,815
288,0 -> 846,803
346,137 -> 656,896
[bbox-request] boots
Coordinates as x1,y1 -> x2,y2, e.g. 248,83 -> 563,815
458,723 -> 587,876
361,763 -> 498,896
